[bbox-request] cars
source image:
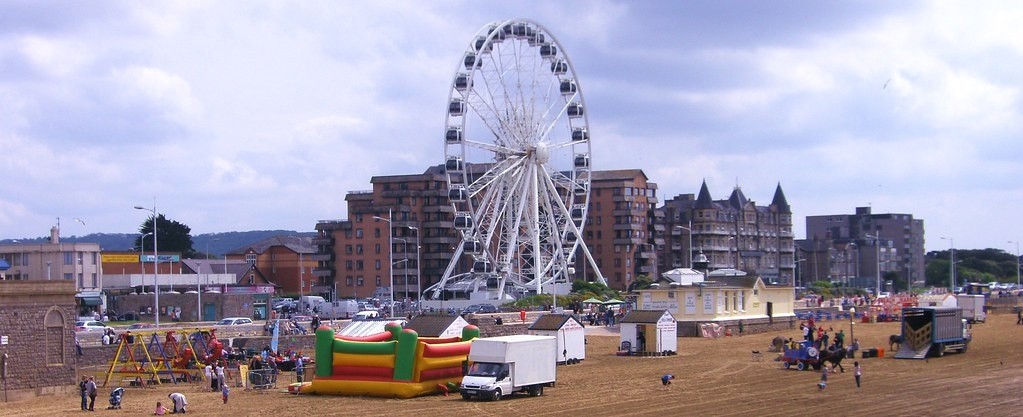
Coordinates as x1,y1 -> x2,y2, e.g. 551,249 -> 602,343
800,293 -> 818,300
879,291 -> 891,299
952,279 -> 1023,298
115,313 -> 140,323
217,316 -> 254,326
75,320 -> 115,332
127,323 -> 154,332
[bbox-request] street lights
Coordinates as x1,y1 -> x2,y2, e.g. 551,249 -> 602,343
47,260 -> 52,282
195,261 -> 203,322
333,281 -> 340,324
793,258 -> 807,288
140,232 -> 154,294
407,225 -> 421,311
675,220 -> 693,270
205,237 -> 222,290
866,230 -> 881,297
643,242 -> 656,283
134,206 -> 159,330
12,239 -> 24,282
1006,239 -> 1021,288
371,207 -> 395,318
393,237 -> 409,308
286,235 -> 304,314
940,236 -> 954,294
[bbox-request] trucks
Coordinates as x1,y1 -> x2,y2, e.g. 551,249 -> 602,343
894,306 -> 973,359
956,294 -> 988,323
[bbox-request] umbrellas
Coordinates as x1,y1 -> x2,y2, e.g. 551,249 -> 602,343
582,297 -> 624,311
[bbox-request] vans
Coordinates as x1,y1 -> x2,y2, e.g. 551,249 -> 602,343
352,310 -> 379,321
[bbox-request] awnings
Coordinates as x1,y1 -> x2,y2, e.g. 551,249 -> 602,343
81,297 -> 103,305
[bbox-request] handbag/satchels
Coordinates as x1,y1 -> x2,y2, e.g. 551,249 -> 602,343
90,383 -> 97,397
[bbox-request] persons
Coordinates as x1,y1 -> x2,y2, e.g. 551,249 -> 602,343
90,310 -> 109,321
79,375 -> 98,412
795,293 -> 801,300
74,332 -> 84,356
367,298 -> 453,320
569,299 -> 636,327
491,315 -> 503,325
153,393 -> 188,415
853,361 -> 862,388
266,306 -> 322,335
102,328 -> 134,345
820,364 -> 828,383
511,303 -> 552,312
201,342 -> 303,392
805,293 -> 877,307
784,313 -> 860,360
170,309 -> 182,322
1017,309 -> 1023,325
661,374 -> 675,391
222,385 -> 229,404
